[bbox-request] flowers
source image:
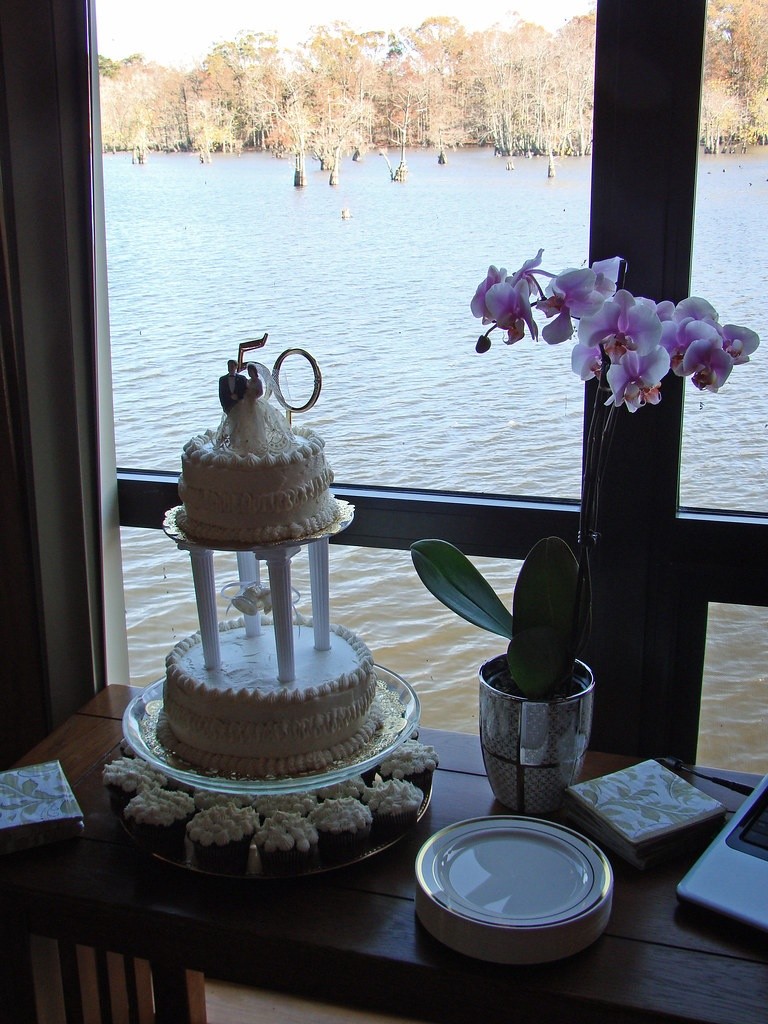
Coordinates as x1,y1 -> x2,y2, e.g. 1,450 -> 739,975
471,248 -> 760,415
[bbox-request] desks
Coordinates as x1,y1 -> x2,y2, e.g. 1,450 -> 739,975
0,683 -> 768,1024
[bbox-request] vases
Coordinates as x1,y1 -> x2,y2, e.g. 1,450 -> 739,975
479,650 -> 596,815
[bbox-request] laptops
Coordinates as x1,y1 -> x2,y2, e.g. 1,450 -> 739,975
673,774 -> 767,932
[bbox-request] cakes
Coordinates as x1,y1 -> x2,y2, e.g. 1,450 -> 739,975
177,333 -> 336,543
164,617 -> 381,772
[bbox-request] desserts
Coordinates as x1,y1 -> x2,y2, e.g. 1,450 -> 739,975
106,734 -> 438,873
